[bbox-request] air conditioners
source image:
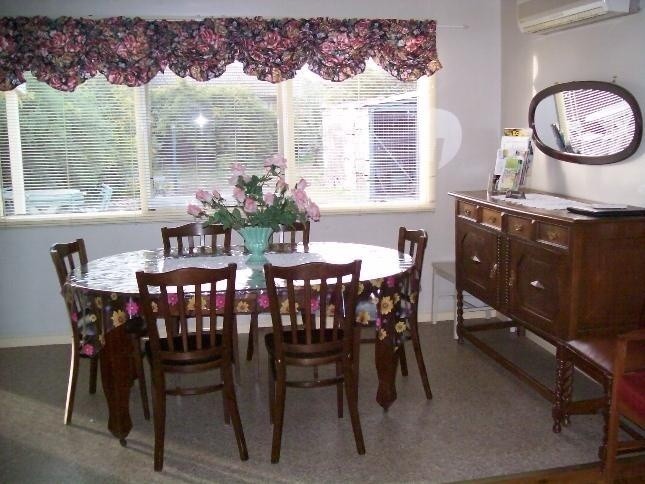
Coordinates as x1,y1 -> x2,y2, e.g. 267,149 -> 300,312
515,0 -> 641,36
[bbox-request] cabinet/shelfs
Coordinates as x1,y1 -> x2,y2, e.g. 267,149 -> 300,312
449,188 -> 644,434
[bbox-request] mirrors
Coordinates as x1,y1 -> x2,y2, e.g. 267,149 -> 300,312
528,81 -> 642,165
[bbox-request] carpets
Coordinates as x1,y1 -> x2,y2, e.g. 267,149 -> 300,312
0,315 -> 644,484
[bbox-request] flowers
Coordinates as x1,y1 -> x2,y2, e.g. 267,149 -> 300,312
186,155 -> 321,234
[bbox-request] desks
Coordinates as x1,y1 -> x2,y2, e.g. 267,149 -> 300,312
60,240 -> 420,447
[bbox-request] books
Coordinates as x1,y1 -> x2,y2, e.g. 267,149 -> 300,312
494,127 -> 534,192
566,203 -> 644,216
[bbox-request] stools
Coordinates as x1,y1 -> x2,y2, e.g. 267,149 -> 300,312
552,334 -> 617,474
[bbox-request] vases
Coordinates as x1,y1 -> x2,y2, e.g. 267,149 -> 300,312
235,227 -> 274,266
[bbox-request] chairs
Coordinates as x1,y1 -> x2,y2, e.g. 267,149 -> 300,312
160,221 -> 241,386
432,261 -> 516,343
263,259 -> 366,464
245,220 -> 310,361
135,263 -> 248,472
50,237 -> 150,426
602,330 -> 644,484
350,226 -> 433,408
